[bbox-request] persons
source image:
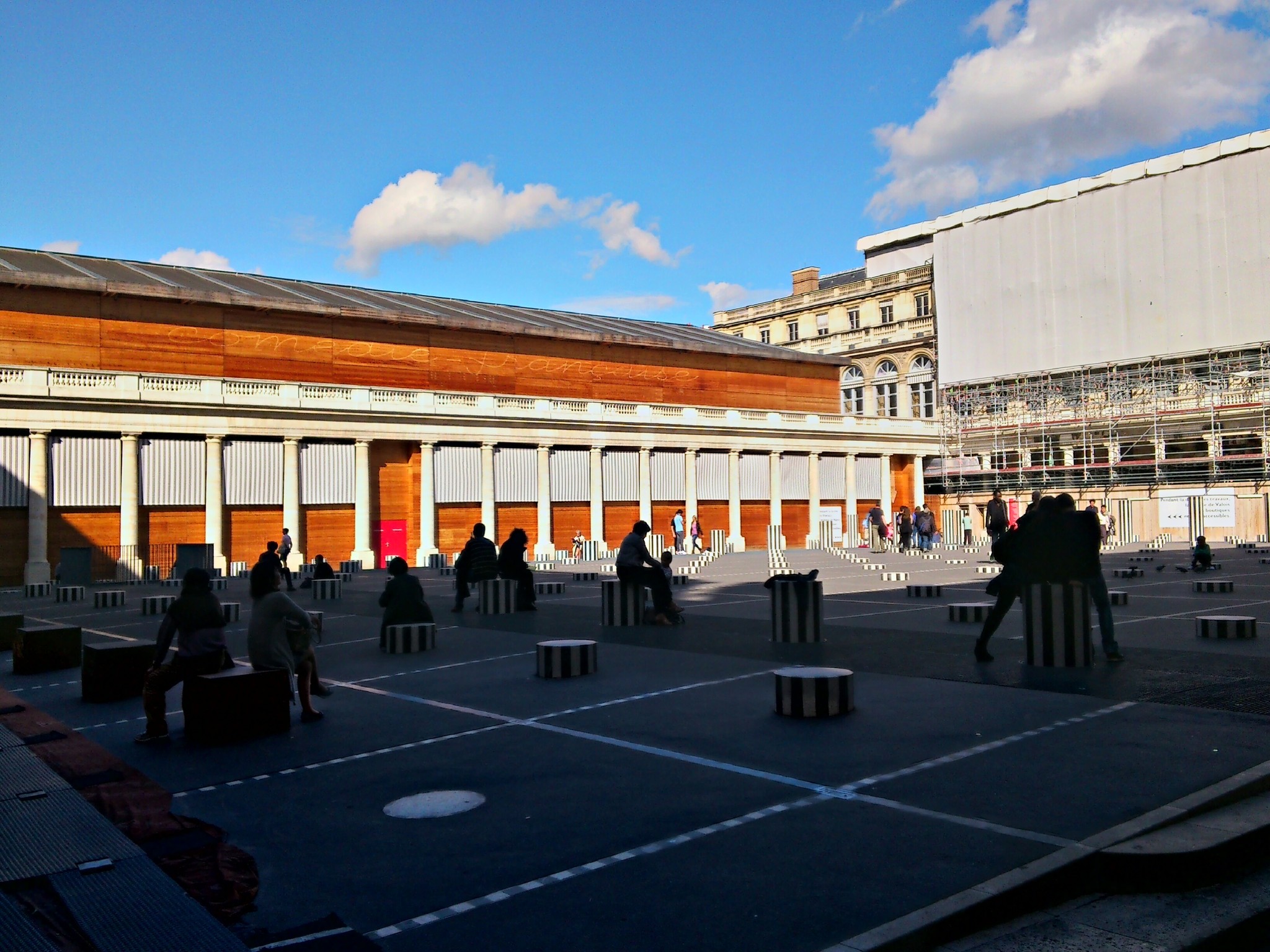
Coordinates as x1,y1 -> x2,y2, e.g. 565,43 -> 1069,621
615,488 -> 1211,663
134,522 -> 536,740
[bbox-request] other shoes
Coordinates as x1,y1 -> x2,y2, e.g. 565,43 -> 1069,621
912,546 -> 916,548
875,552 -> 878,554
455,605 -> 463,612
917,546 -> 920,548
300,712 -> 323,720
310,687 -> 332,697
881,549 -> 886,553
975,638 -> 993,662
287,586 -> 295,591
134,731 -> 169,741
1107,652 -> 1124,661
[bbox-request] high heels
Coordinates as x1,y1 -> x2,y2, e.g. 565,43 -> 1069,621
664,604 -> 684,614
654,616 -> 672,626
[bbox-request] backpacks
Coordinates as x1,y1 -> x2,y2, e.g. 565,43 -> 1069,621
920,516 -> 931,533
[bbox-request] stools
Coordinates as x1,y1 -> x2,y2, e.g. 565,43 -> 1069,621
0,553 -> 458,758
480,522 -> 1270,738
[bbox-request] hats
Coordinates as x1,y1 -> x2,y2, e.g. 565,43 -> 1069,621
923,507 -> 931,513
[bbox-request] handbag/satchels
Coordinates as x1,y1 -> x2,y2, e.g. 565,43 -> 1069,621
277,547 -> 285,554
300,580 -> 312,589
668,612 -> 685,624
932,532 -> 940,543
878,524 -> 888,541
